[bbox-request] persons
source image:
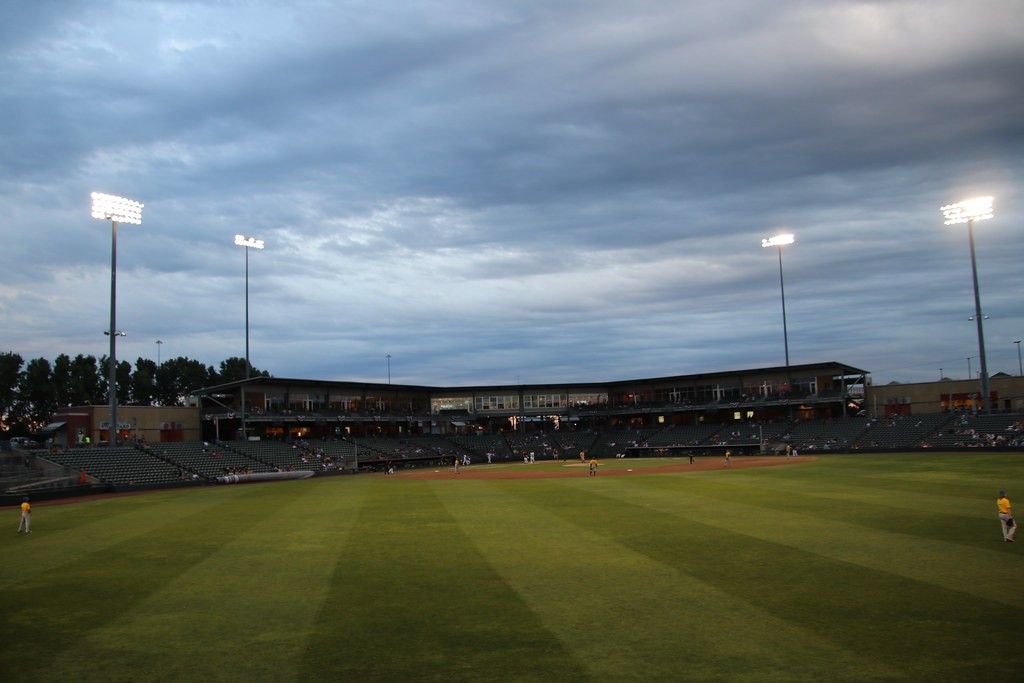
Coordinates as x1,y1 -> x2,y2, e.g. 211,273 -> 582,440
786,443 -> 798,457
80,468 -> 86,483
17,497 -> 33,533
488,451 -> 491,464
724,449 -> 730,466
508,429 -> 578,461
689,450 -> 694,464
178,469 -> 182,476
462,454 -> 471,466
454,458 -> 460,474
580,449 -> 586,463
952,411 -> 1024,447
522,451 -> 535,464
384,459 -> 394,474
201,436 -> 462,488
192,472 -> 199,479
997,490 -> 1017,541
85,436 -> 90,449
589,457 -> 597,476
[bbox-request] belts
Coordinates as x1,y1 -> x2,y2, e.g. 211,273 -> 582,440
999,512 -> 1007,514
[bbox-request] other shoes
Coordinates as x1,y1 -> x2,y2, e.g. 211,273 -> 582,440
1007,537 -> 1015,542
1004,538 -> 1008,542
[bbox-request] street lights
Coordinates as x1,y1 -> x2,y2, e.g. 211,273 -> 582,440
940,195 -> 995,415
235,234 -> 264,379
91,192 -> 144,448
386,353 -> 391,384
1013,340 -> 1022,376
155,340 -> 163,369
762,234 -> 794,366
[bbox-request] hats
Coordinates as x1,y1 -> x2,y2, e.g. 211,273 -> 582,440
1000,489 -> 1006,494
